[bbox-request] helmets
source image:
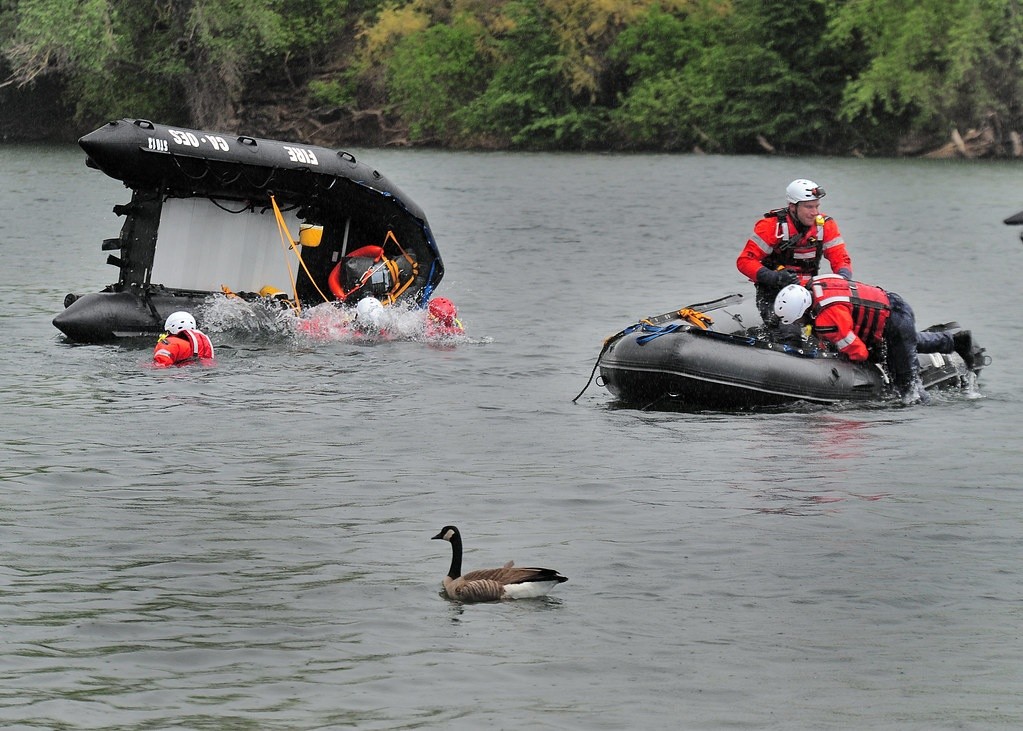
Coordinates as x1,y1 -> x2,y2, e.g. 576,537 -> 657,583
785,178 -> 826,205
356,297 -> 383,327
163,310 -> 196,335
774,284 -> 814,325
427,297 -> 457,327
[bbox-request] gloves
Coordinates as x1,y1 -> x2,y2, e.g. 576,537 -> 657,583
838,269 -> 853,282
757,267 -> 800,289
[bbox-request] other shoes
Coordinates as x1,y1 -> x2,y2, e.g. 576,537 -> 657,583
953,329 -> 973,371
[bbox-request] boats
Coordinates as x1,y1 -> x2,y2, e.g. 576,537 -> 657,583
52,116 -> 446,341
595,291 -> 961,410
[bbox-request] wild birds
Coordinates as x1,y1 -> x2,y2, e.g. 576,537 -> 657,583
431,525 -> 569,603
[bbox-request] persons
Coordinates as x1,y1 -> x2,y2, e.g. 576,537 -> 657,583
774,278 -> 974,398
736,179 -> 854,343
152,311 -> 214,368
426,297 -> 463,338
296,297 -> 394,342
